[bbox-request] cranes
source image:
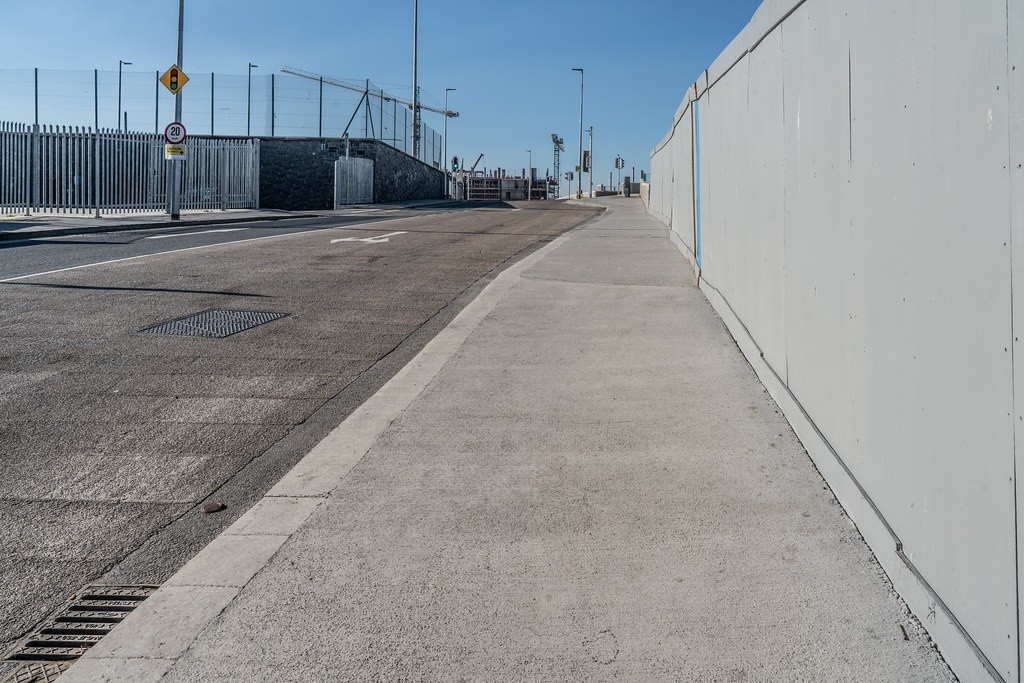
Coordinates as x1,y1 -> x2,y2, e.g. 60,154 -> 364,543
279,66 -> 460,161
551,133 -> 566,199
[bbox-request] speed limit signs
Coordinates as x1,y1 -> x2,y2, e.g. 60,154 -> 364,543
165,122 -> 187,144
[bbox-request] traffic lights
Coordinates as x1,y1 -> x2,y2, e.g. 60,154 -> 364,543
546,177 -> 550,184
452,157 -> 459,170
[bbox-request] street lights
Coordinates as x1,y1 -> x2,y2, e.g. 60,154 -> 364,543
526,150 -> 531,200
445,88 -> 456,198
248,62 -> 258,136
584,126 -> 593,198
118,60 -> 133,132
571,67 -> 585,199
